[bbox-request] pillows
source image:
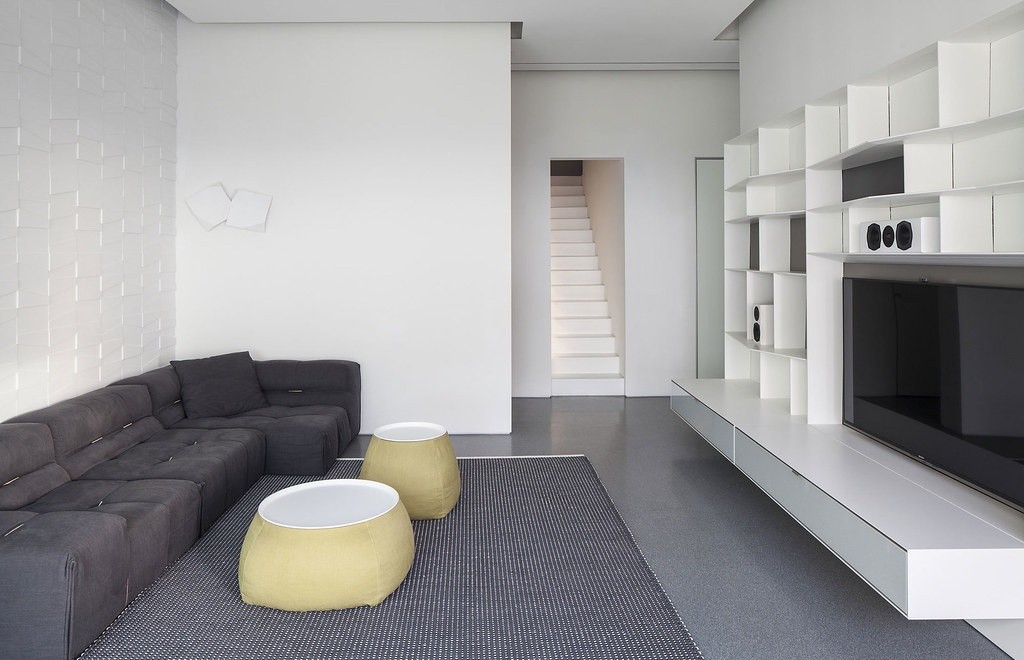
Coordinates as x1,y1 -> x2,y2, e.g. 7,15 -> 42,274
170,351 -> 269,419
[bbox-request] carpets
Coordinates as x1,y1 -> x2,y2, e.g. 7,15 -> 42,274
74,454 -> 707,660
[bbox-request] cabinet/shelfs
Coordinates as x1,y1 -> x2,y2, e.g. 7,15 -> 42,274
724,29 -> 1024,426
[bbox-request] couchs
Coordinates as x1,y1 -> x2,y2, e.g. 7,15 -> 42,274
0,358 -> 361,660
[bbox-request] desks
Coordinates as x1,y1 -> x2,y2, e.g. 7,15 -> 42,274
362,421 -> 461,520
239,479 -> 415,612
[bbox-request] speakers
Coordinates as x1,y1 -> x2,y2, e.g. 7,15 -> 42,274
858,217 -> 940,253
752,304 -> 774,344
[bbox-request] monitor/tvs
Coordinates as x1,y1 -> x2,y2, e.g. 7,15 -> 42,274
842,276 -> 1024,513
